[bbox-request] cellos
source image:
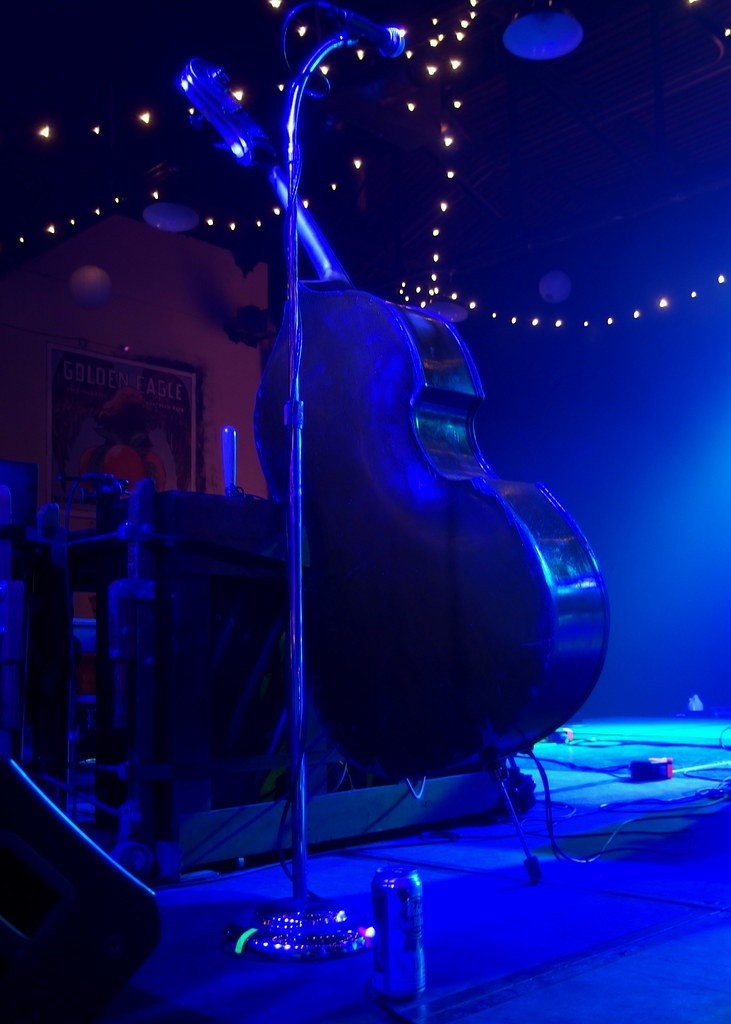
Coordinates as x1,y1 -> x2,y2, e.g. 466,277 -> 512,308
178,58 -> 609,879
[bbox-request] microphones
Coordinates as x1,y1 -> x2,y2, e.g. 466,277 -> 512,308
317,2 -> 404,60
